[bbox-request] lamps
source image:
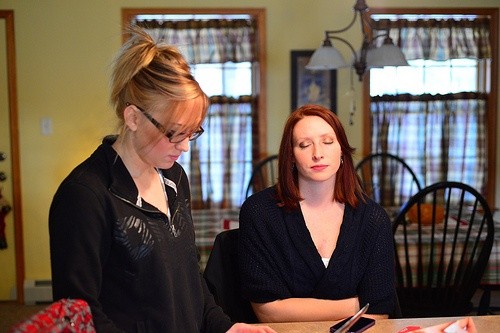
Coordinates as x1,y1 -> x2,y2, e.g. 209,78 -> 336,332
305,0 -> 411,126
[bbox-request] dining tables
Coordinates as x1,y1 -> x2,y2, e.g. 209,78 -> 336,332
188,201 -> 500,315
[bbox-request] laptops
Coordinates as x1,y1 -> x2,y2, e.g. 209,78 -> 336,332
334,303 -> 369,333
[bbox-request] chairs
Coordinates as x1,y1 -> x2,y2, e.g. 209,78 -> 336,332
355,152 -> 422,207
392,181 -> 494,318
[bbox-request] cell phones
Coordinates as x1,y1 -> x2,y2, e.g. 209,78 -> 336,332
330,316 -> 375,333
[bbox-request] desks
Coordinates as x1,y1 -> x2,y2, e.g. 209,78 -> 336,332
246,315 -> 500,333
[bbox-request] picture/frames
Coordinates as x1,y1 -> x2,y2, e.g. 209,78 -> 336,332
290,49 -> 337,117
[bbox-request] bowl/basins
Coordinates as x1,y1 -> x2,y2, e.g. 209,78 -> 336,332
405,204 -> 444,225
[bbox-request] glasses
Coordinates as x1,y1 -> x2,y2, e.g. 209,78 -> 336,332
126,102 -> 204,143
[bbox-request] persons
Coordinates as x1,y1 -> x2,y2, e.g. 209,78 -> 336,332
238,104 -> 396,322
49,28 -> 276,333
